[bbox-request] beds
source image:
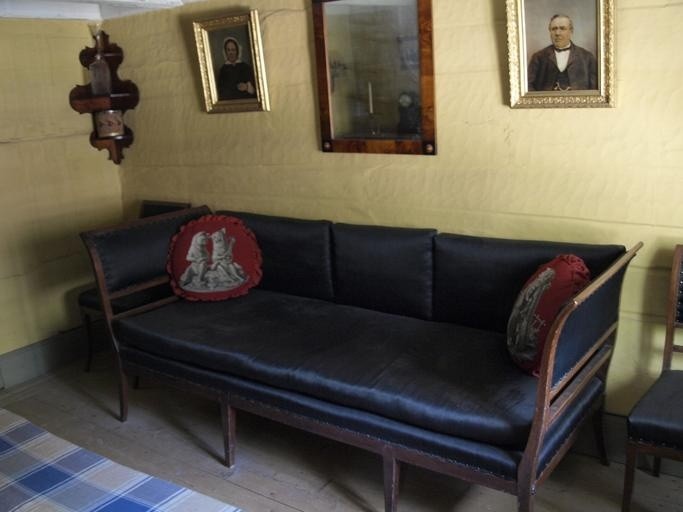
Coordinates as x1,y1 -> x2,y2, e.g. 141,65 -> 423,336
1,406 -> 242,510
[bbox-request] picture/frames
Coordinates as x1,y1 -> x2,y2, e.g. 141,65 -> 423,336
503,0 -> 617,109
189,8 -> 272,114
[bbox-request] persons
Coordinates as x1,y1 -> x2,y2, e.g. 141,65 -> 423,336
526,13 -> 597,92
215,36 -> 255,102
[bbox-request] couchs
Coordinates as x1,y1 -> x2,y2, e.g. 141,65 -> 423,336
75,205 -> 645,510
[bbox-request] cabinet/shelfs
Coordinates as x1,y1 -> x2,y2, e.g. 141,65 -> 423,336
71,30 -> 141,163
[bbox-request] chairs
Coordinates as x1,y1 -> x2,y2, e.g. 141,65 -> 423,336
618,242 -> 682,510
62,200 -> 214,394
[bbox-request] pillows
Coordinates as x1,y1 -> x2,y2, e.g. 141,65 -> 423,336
499,251 -> 592,374
166,214 -> 264,299
